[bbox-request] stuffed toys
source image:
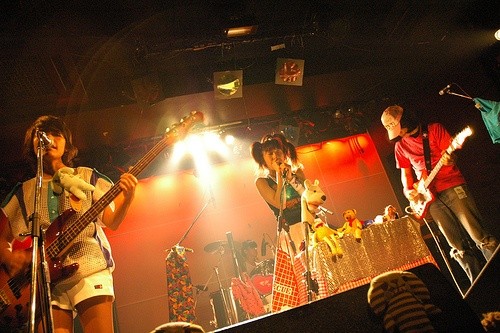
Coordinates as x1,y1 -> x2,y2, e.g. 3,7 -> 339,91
312,213 -> 344,263
335,208 -> 362,242
50,166 -> 95,201
301,176 -> 326,227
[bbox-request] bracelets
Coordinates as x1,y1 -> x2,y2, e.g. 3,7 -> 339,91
288,175 -> 302,191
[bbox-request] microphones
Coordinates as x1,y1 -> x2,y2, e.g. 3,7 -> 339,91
195,285 -> 209,291
260,238 -> 267,256
38,131 -> 54,149
438,84 -> 452,95
276,158 -> 286,173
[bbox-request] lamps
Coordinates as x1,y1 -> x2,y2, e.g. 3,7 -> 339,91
206,44 -> 245,101
274,56 -> 305,86
223,16 -> 258,37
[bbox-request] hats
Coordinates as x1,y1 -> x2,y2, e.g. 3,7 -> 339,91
381,105 -> 403,140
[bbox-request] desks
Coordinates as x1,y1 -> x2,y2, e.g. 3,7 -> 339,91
292,218 -> 435,304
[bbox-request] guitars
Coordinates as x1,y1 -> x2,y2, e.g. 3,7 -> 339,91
410,125 -> 473,220
0,111 -> 205,333
226,231 -> 266,316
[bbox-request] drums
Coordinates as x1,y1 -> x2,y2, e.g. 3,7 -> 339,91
210,287 -> 247,329
197,288 -> 247,332
249,259 -> 275,299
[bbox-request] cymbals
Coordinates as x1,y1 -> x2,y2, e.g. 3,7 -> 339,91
204,241 -> 241,255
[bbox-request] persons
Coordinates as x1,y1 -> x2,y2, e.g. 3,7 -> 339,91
0,114 -> 138,333
240,239 -> 260,276
250,133 -> 329,302
381,105 -> 498,286
384,204 -> 399,219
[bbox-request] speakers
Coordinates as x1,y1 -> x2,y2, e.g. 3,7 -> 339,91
464,243 -> 500,333
205,264 -> 486,333
